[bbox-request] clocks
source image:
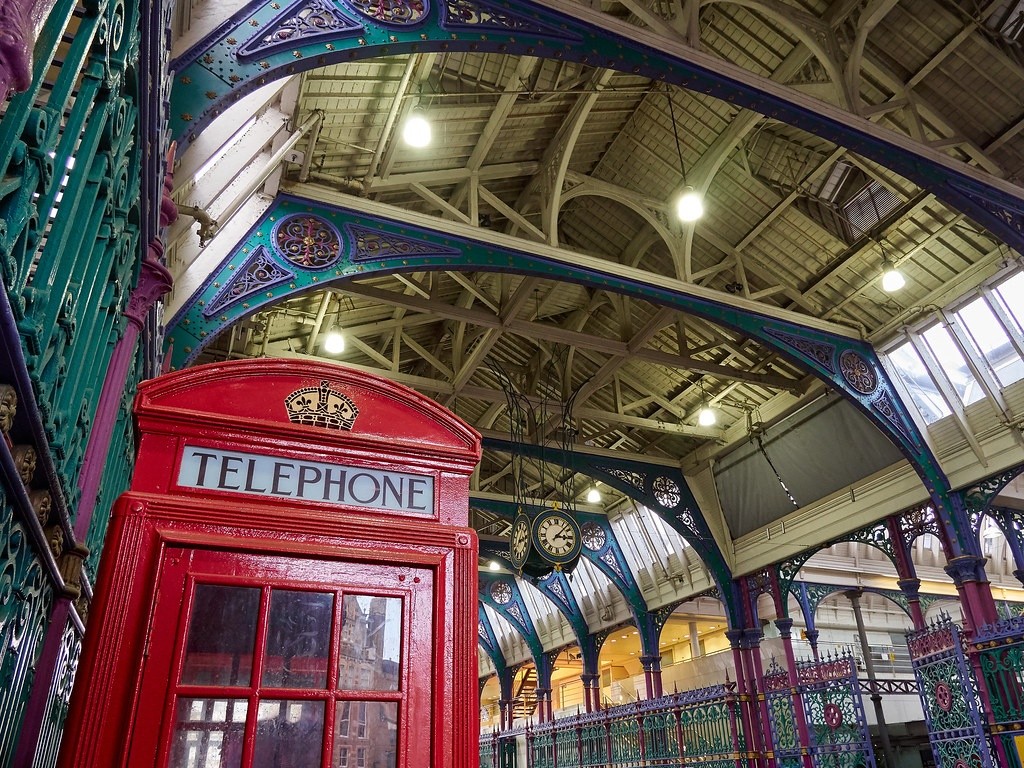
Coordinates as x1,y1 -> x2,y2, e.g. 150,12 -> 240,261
531,509 -> 583,564
509,512 -> 532,570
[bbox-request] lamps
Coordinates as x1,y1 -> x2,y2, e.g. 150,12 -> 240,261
698,378 -> 716,426
666,83 -> 704,221
403,84 -> 432,147
868,228 -> 906,291
324,298 -> 345,353
587,477 -> 600,503
